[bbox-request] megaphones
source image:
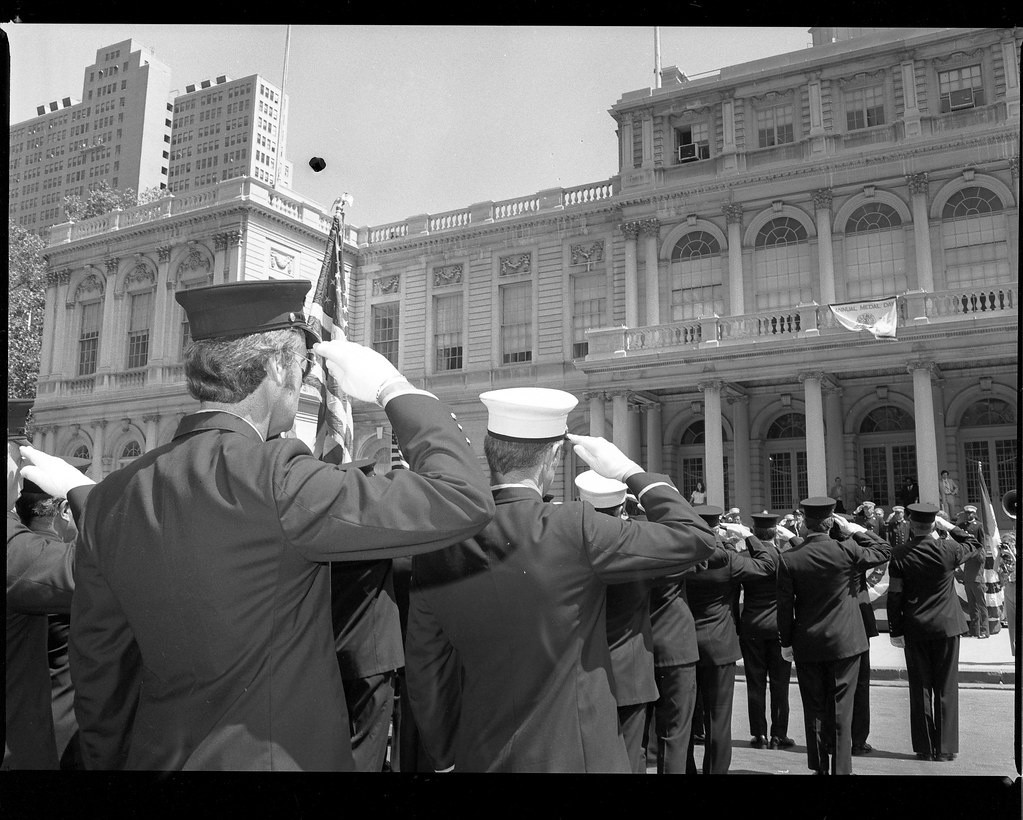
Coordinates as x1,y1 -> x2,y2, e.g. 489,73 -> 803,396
1002,490 -> 1017,521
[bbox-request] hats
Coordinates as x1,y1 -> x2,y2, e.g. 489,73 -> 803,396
574,469 -> 629,509
21,456 -> 92,493
478,387 -> 574,443
6,399 -> 34,440
334,458 -> 380,478
175,280 -> 323,350
694,496 -> 981,528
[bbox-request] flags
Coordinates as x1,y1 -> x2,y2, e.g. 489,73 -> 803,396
976,467 -> 1003,632
301,200 -> 355,470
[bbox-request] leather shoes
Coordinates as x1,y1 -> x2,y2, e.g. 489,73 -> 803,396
693,734 -> 705,745
852,742 -> 872,755
936,753 -> 957,761
750,735 -> 768,750
770,736 -> 795,751
915,753 -> 934,761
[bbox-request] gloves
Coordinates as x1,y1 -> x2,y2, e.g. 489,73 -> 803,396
935,516 -> 956,532
18,446 -> 97,500
313,340 -> 409,407
889,635 -> 905,648
774,525 -> 795,542
850,522 -> 867,534
780,646 -> 795,662
720,523 -> 753,540
565,432 -> 636,485
832,512 -> 854,536
853,505 -> 863,515
627,493 -> 645,512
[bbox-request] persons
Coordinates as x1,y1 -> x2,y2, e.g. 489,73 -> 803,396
399,386 -> 718,774
6,398 -> 99,771
569,468 -> 1016,774
14,454 -> 81,774
66,277 -> 492,771
329,458 -> 406,773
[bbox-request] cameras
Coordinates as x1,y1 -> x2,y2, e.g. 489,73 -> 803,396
1000,542 -> 1010,549
